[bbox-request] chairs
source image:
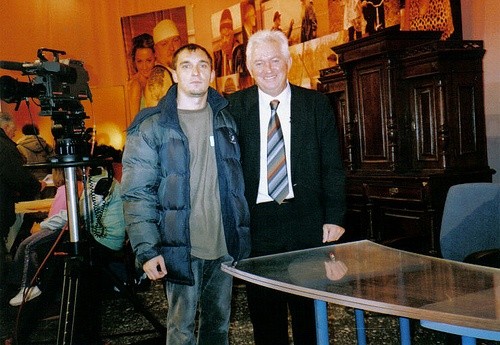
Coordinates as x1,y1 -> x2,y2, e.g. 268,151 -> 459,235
439,182 -> 500,262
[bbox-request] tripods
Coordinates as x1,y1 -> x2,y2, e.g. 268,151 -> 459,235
22,116 -> 167,345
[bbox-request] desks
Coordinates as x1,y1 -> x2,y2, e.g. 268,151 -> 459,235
221,239 -> 500,345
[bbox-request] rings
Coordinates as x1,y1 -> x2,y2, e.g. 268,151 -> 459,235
336,231 -> 340,235
152,271 -> 158,275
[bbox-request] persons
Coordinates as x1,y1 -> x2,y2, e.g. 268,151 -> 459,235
232,257 -> 348,289
130,0 -> 385,120
226,29 -> 348,345
0,124 -> 130,306
121,43 -> 252,345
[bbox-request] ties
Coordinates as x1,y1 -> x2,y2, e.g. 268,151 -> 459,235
267,99 -> 290,205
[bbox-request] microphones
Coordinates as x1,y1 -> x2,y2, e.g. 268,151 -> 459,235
0,61 -> 31,72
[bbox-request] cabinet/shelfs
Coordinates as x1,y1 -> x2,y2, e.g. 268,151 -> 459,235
318,24 -> 497,258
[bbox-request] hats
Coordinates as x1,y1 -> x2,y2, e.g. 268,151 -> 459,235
222,9 -> 234,32
273,12 -> 281,22
152,19 -> 180,44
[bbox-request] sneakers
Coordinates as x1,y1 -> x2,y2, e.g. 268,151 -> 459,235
9,285 -> 42,307
